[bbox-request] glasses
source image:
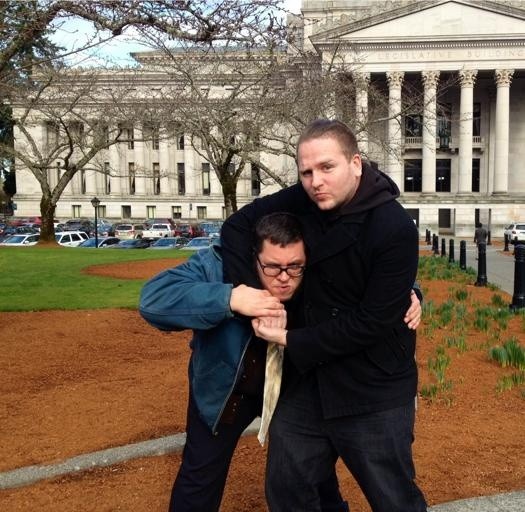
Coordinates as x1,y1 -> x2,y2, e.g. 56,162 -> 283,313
257,256 -> 309,279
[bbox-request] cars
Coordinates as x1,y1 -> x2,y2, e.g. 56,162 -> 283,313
504,222 -> 525,245
0,216 -> 224,250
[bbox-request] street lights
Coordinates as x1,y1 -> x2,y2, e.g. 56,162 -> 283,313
405,177 -> 414,192
91,197 -> 100,248
438,176 -> 444,192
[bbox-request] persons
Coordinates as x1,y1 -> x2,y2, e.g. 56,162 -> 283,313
216,118 -> 431,511
473,222 -> 488,261
136,206 -> 425,510
